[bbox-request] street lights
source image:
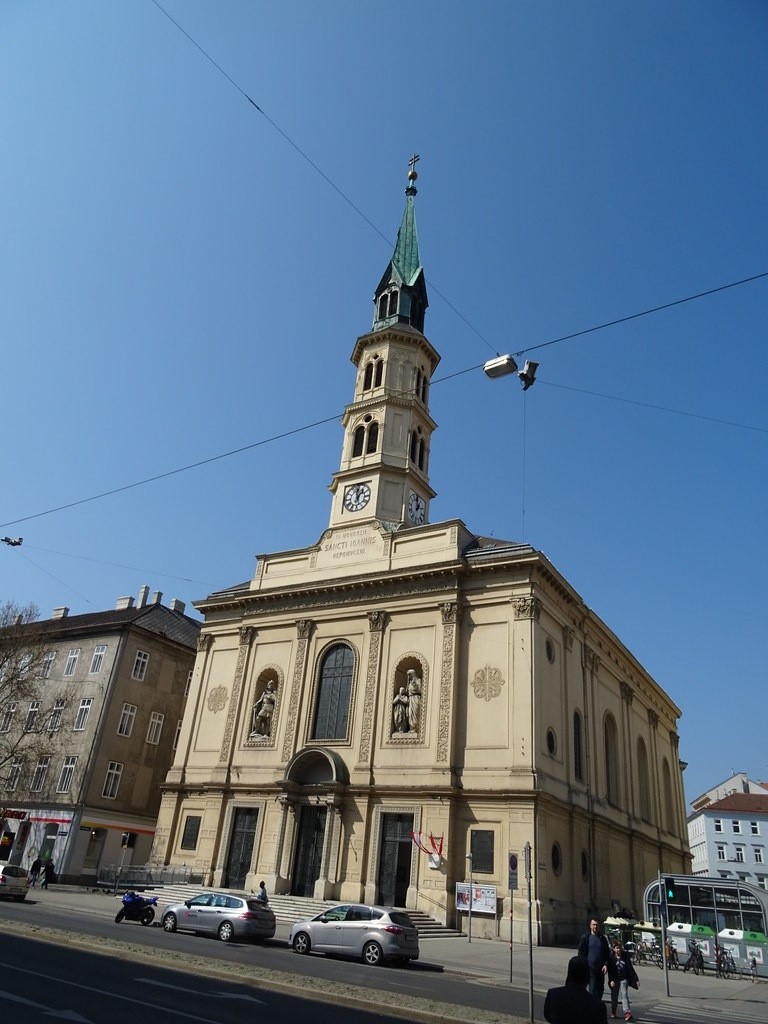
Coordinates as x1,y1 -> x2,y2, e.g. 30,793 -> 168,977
465,853 -> 472,943
114,833 -> 131,895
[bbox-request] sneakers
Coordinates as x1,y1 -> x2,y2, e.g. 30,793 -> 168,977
625,1012 -> 632,1021
610,1012 -> 616,1019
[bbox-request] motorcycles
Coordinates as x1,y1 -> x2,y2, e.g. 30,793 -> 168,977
114,889 -> 159,925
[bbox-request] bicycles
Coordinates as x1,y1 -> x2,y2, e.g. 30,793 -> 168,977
604,928 -> 759,984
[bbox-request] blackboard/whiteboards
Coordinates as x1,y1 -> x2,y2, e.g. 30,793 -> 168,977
454,881 -> 497,914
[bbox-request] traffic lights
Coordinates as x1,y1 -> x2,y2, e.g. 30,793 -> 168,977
664,877 -> 676,902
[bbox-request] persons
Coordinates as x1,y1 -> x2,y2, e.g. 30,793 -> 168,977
260,880 -> 267,897
28,855 -> 56,890
542,957 -> 607,1023
250,680 -> 277,739
577,918 -> 641,1021
393,668 -> 423,733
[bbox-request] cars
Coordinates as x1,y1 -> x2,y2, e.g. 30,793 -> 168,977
161,893 -> 276,942
288,903 -> 419,966
0,865 -> 31,900
